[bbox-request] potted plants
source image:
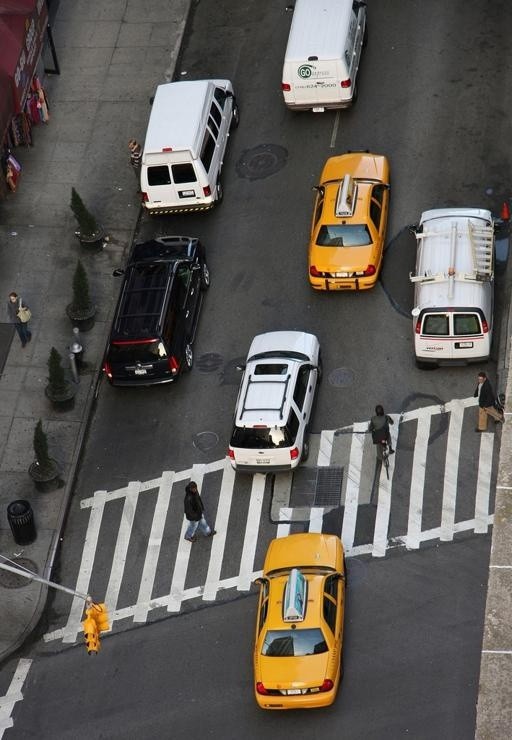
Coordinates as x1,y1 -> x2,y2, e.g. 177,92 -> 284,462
27,188 -> 106,494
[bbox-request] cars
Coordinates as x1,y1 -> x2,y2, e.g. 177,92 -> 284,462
307,149 -> 391,294
102,234 -> 210,389
251,531 -> 349,713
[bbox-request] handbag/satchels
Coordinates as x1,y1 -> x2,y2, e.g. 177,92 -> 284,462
17,298 -> 32,323
372,428 -> 388,445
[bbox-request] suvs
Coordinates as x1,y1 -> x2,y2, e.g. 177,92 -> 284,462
226,329 -> 322,475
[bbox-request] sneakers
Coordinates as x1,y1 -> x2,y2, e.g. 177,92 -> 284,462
185,538 -> 196,542
474,429 -> 487,432
208,531 -> 217,537
376,456 -> 382,463
22,331 -> 32,348
495,421 -> 502,424
388,446 -> 395,454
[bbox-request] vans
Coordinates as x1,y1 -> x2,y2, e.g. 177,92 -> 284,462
408,206 -> 497,371
138,77 -> 240,220
281,0 -> 368,115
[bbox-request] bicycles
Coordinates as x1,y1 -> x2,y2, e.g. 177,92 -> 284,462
379,440 -> 390,481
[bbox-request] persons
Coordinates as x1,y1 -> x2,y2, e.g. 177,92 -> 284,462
128,139 -> 144,179
184,480 -> 216,543
474,371 -> 505,433
6,291 -> 32,348
369,404 -> 396,461
